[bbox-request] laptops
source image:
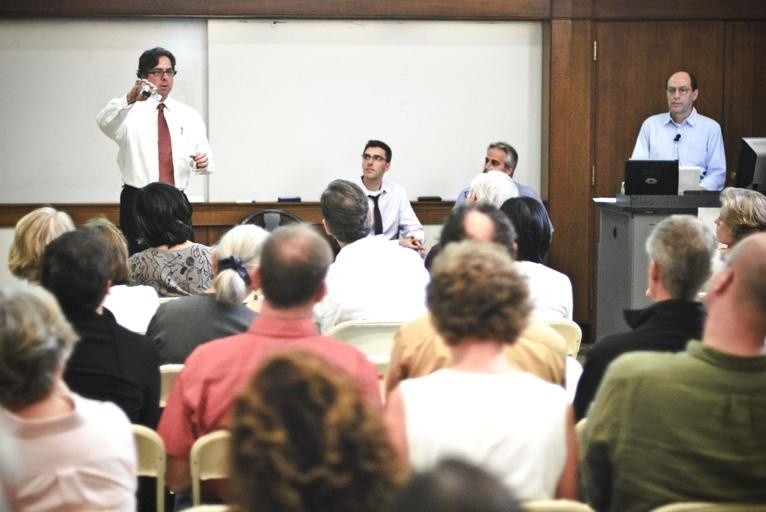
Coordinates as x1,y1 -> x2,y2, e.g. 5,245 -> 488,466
625,160 -> 678,194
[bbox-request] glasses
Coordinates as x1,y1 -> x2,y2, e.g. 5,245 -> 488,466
666,86 -> 692,95
146,69 -> 177,78
361,154 -> 389,161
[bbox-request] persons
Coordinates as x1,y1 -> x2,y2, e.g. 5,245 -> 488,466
0,141 -> 765,512
621,69 -> 726,192
96,47 -> 209,255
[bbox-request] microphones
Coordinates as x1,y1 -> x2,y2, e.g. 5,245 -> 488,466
674,134 -> 681,141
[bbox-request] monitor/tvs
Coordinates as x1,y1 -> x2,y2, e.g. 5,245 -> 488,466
735,136 -> 766,194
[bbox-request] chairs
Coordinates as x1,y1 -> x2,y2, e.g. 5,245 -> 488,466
241,209 -> 300,229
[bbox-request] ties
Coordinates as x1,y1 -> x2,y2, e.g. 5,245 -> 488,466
368,194 -> 383,236
156,103 -> 175,187
